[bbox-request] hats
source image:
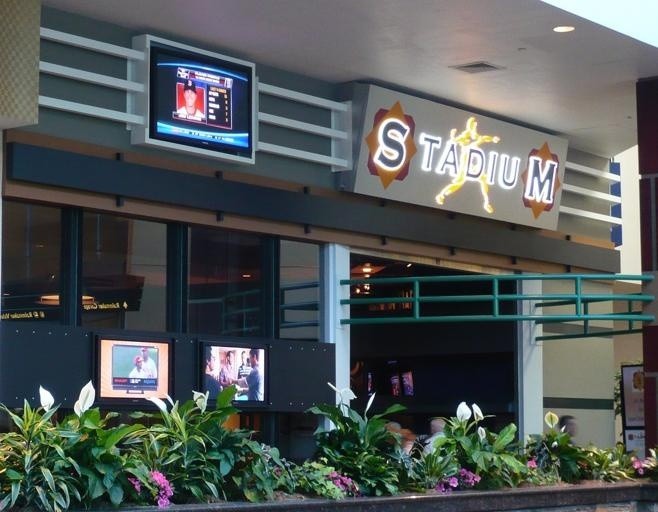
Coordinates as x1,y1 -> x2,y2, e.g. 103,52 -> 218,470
184,80 -> 196,93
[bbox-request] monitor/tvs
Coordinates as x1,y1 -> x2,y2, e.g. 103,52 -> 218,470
197,339 -> 273,408
96,333 -> 173,406
130,34 -> 256,167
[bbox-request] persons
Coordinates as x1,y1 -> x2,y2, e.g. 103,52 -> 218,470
227,349 -> 263,401
218,350 -> 237,400
351,354 -> 366,415
200,345 -> 224,400
139,347 -> 157,380
128,356 -> 152,382
174,80 -> 208,122
425,417 -> 447,453
234,351 -> 252,379
557,412 -> 581,446
